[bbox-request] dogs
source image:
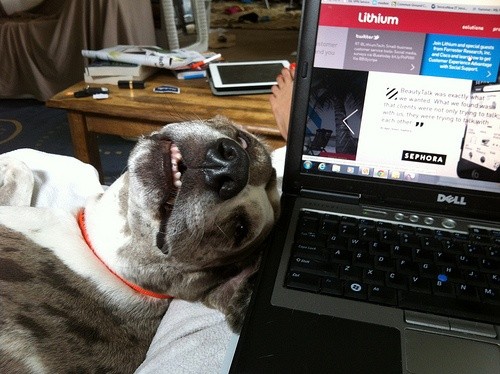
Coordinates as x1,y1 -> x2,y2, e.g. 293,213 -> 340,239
0,113 -> 282,374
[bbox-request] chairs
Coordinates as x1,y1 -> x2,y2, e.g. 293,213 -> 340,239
304,129 -> 333,156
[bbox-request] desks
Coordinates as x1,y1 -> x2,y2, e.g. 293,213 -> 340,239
46,73 -> 287,185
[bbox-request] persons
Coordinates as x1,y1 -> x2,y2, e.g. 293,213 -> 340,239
269,67 -> 297,142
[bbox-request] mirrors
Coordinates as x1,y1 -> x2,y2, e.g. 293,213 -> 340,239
152,0 -> 208,53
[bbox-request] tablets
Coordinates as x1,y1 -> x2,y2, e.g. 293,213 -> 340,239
210,59 -> 293,90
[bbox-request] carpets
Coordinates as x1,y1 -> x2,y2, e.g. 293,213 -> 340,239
209,0 -> 302,30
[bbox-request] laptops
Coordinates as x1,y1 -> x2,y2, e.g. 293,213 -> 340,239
228,0 -> 500,374
